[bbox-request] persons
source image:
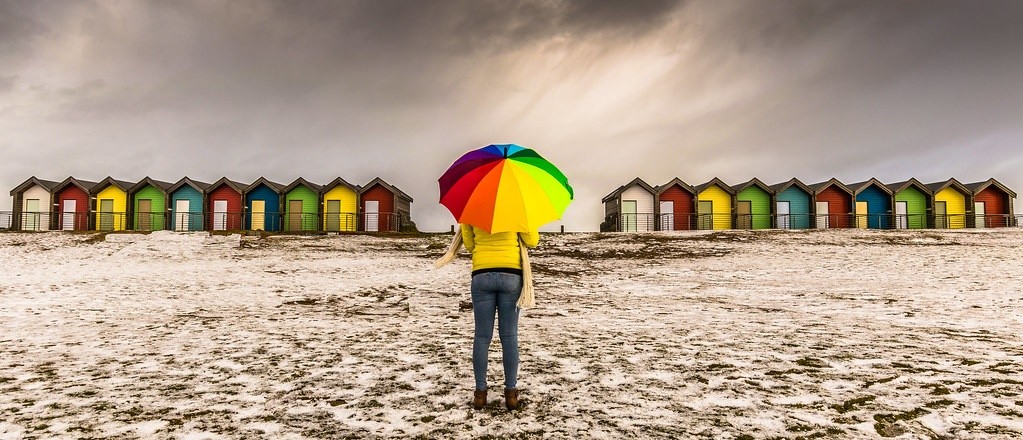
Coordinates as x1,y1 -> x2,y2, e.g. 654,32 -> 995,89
433,222 -> 539,410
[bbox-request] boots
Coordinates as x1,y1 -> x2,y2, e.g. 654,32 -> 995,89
473,389 -> 487,411
505,388 -> 518,410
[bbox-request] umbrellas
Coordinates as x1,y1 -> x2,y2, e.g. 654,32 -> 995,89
437,144 -> 574,237
